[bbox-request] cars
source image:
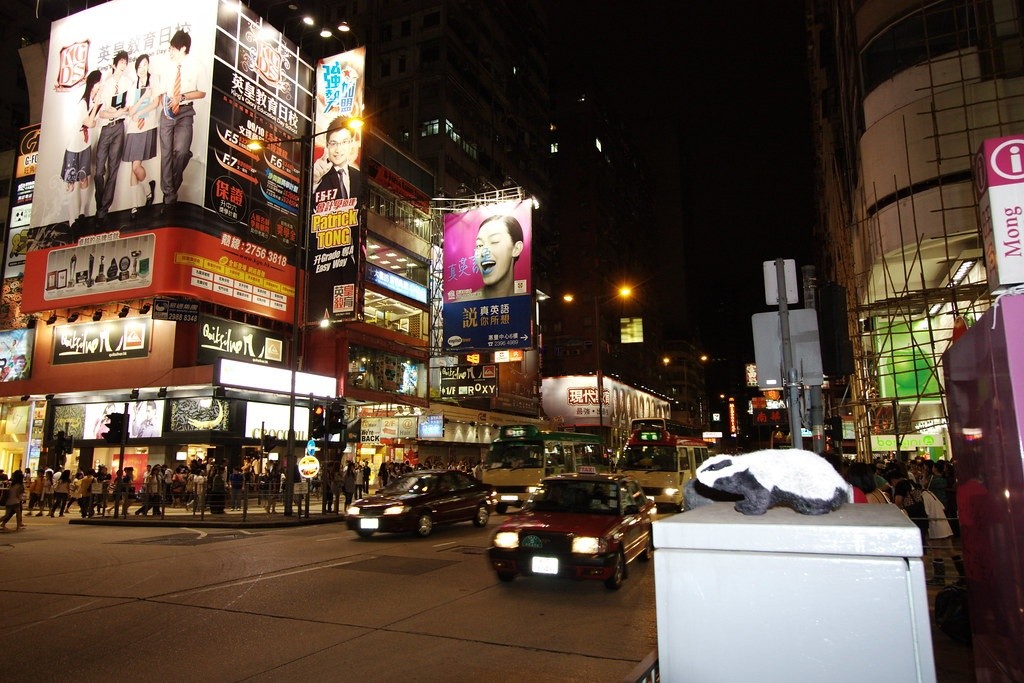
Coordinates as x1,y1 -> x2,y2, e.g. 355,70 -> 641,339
487,466 -> 657,591
343,469 -> 498,538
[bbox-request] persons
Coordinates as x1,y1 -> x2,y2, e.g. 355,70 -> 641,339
0,340 -> 27,382
543,484 -> 616,510
501,448 -> 519,464
24,458 -> 370,518
61,29 -> 206,233
377,458 -> 486,493
0,465 -> 25,533
93,401 -> 160,439
452,215 -> 524,302
821,451 -> 964,585
557,450 -> 565,465
629,446 -> 669,468
310,116 -> 361,235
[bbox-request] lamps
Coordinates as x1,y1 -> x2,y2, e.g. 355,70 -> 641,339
318,27 -> 347,52
477,176 -> 497,191
456,183 -> 476,195
266,0 -> 300,22
437,186 -> 454,198
130,388 -> 140,400
46,394 -> 55,402
282,15 -> 314,37
46,314 -> 68,325
67,312 -> 92,323
27,315 -> 48,329
157,387 -> 167,398
337,21 -> 359,46
20,394 -> 30,404
93,309 -> 116,322
138,303 -> 154,316
214,386 -> 226,398
117,305 -> 139,319
502,175 -> 519,187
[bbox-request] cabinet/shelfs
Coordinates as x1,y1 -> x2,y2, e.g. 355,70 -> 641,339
376,355 -> 398,391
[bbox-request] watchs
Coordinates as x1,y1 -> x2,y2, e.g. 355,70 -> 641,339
180,94 -> 186,103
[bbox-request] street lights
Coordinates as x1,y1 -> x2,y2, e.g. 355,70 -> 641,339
562,286 -> 632,438
249,119 -> 364,517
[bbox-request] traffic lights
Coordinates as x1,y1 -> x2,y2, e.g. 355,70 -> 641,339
312,405 -> 325,438
327,401 -> 347,435
101,412 -> 121,442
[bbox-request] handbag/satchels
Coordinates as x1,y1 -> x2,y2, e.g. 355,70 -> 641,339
903,479 -> 924,514
87,484 -> 92,492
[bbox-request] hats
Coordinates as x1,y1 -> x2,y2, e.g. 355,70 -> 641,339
35,469 -> 45,474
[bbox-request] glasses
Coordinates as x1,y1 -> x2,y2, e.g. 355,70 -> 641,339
328,138 -> 353,146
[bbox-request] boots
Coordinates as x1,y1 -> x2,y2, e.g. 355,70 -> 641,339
925,558 -> 945,587
943,554 -> 968,592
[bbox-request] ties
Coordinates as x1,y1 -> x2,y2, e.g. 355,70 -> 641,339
338,168 -> 348,199
115,84 -> 119,96
172,64 -> 181,115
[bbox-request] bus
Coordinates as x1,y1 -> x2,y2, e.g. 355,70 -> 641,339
614,434 -> 712,512
481,424 -> 609,515
632,417 -> 704,438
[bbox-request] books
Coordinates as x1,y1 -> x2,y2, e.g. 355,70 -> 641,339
163,94 -> 176,121
133,87 -> 150,118
109,91 -> 127,120
80,103 -> 103,132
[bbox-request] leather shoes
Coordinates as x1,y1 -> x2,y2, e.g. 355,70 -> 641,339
68,214 -> 88,236
160,202 -> 169,216
90,211 -> 108,228
146,180 -> 155,207
129,208 -> 139,219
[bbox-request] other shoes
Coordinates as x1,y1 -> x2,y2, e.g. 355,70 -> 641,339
237,507 -> 240,511
36,513 -> 42,516
231,508 -> 234,511
25,513 -> 31,516
